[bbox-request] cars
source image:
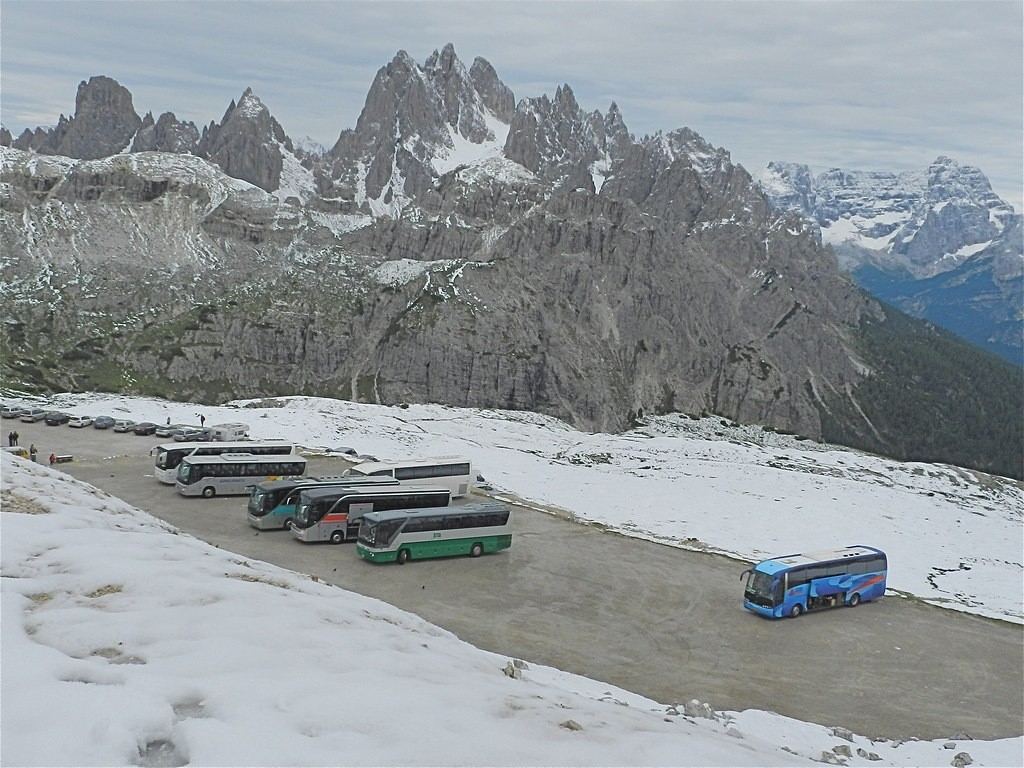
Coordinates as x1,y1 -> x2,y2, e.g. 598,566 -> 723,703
155,424 -> 179,437
93,416 -> 116,428
1,405 -> 24,418
67,415 -> 93,428
20,407 -> 48,423
134,422 -> 157,436
44,411 -> 70,426
114,419 -> 137,432
174,427 -> 200,443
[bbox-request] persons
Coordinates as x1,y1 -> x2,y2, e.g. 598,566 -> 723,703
50,454 -> 55,465
201,415 -> 205,427
8,431 -> 19,446
167,417 -> 171,424
30,444 -> 38,454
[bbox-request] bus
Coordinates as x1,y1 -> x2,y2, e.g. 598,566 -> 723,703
244,475 -> 400,530
287,485 -> 452,544
352,503 -> 512,565
342,455 -> 472,497
149,440 -> 295,484
740,546 -> 887,619
174,452 -> 308,498
